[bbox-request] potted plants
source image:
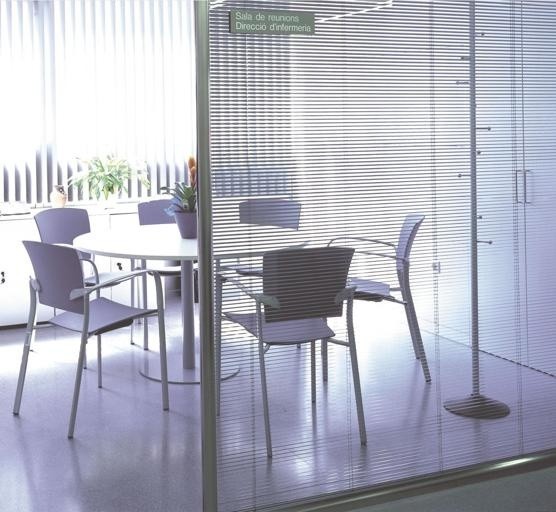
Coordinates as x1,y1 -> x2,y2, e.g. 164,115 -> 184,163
61,155 -> 153,210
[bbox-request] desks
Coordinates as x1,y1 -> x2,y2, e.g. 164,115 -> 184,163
74,219 -> 309,389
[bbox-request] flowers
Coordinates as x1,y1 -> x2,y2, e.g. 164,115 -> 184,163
171,156 -> 202,213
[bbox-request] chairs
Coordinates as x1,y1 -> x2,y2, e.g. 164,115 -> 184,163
319,214 -> 434,386
220,197 -> 304,302
33,205 -> 137,369
15,238 -> 172,439
211,247 -> 372,459
131,198 -> 197,350
